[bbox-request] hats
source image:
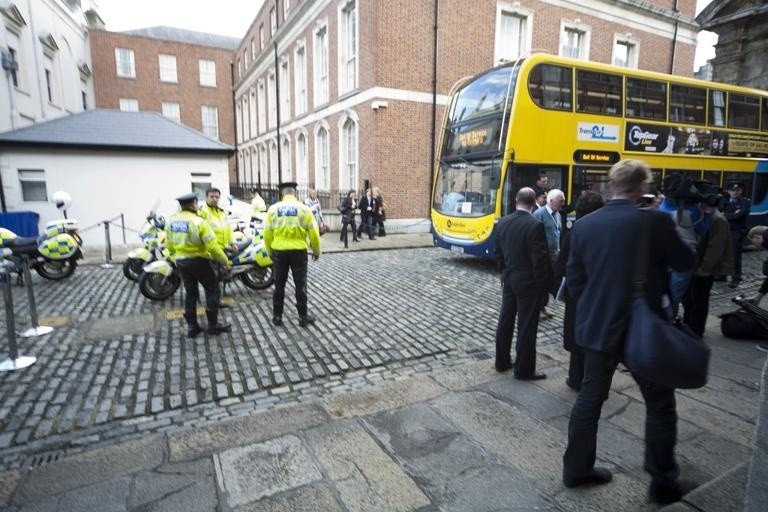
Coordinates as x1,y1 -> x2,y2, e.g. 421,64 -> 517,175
175,192 -> 198,205
727,180 -> 745,190
278,183 -> 298,191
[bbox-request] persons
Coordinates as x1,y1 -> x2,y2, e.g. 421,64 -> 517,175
262,182 -> 321,326
652,172 -> 768,339
166,193 -> 232,337
249,187 -> 266,212
196,188 -> 238,308
357,188 -> 377,241
718,138 -> 727,155
564,159 -> 699,502
489,173 -> 606,392
711,138 -> 718,155
340,190 -> 361,243
304,189 -> 324,247
372,186 -> 386,237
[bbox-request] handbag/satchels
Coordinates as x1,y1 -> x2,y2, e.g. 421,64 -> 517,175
319,225 -> 330,236
717,310 -> 767,340
337,201 -> 348,215
625,297 -> 712,389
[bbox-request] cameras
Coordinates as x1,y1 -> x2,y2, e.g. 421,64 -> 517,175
642,196 -> 656,206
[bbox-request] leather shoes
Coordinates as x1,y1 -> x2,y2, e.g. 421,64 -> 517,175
563,465 -> 612,487
566,376 -> 581,392
650,480 -> 699,504
514,370 -> 546,380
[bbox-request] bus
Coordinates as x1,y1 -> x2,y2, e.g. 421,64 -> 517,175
422,47 -> 767,264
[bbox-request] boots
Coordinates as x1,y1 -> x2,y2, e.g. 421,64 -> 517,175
183,313 -> 203,337
206,310 -> 232,334
271,301 -> 284,326
295,302 -> 314,326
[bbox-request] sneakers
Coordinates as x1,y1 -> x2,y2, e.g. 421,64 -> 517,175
757,343 -> 768,352
539,305 -> 556,318
729,280 -> 738,287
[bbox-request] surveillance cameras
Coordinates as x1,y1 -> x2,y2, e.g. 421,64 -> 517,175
372,103 -> 379,110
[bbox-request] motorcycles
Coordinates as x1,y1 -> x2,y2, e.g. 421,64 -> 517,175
0,189 -> 83,284
122,198 -> 278,301
